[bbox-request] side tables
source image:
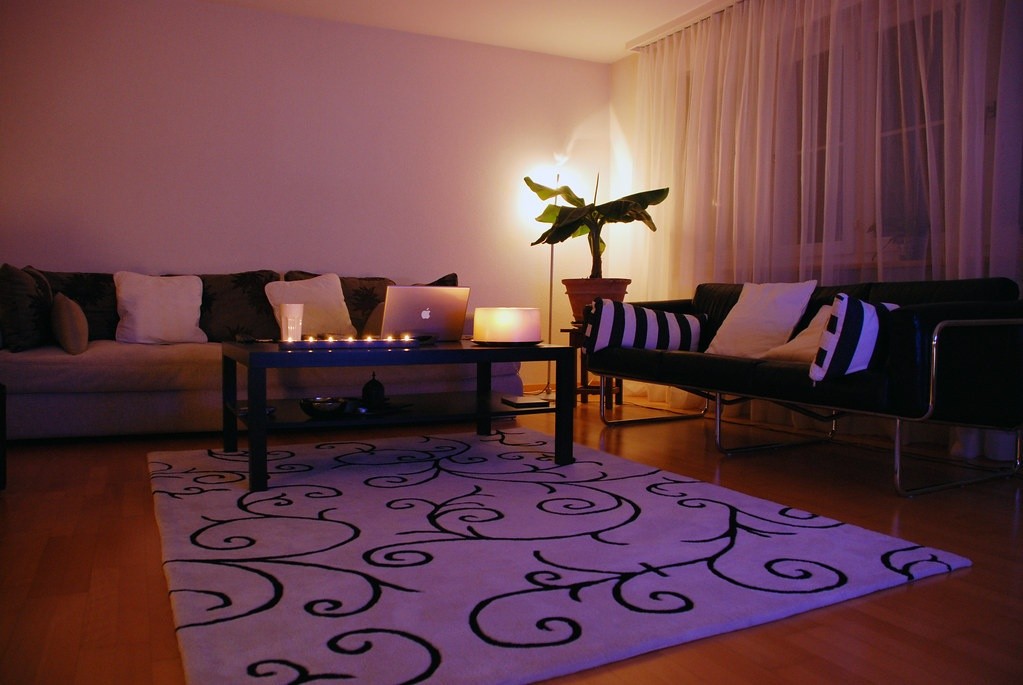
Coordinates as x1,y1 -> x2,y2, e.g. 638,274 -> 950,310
560,328 -> 623,408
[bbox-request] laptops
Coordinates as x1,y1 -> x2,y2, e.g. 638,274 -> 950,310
362,286 -> 471,342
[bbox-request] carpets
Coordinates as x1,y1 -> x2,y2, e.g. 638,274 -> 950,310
147,425 -> 975,685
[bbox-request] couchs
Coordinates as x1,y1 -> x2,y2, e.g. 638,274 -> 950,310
0,270 -> 525,438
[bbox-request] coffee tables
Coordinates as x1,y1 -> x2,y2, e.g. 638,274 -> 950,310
222,340 -> 580,491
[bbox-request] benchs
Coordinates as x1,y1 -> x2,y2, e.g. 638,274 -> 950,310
585,277 -> 1023,495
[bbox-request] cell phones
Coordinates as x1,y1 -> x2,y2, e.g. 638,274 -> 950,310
256,338 -> 274,343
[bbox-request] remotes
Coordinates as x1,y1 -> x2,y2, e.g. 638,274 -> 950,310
236,334 -> 255,345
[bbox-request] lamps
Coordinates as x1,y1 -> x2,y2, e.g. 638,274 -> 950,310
471,307 -> 542,346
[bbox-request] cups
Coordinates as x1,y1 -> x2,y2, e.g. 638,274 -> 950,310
279,303 -> 304,340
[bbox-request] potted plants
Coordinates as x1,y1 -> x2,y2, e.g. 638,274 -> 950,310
523,176 -> 669,329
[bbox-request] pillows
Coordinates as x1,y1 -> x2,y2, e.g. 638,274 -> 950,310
112,270 -> 208,343
760,303 -> 832,363
265,272 -> 358,338
52,292 -> 89,355
430,272 -> 458,286
809,292 -> 900,381
283,270 -> 396,339
200,270 -> 280,343
360,301 -> 384,340
582,296 -> 709,355
705,279 -> 817,358
0,262 -> 57,353
